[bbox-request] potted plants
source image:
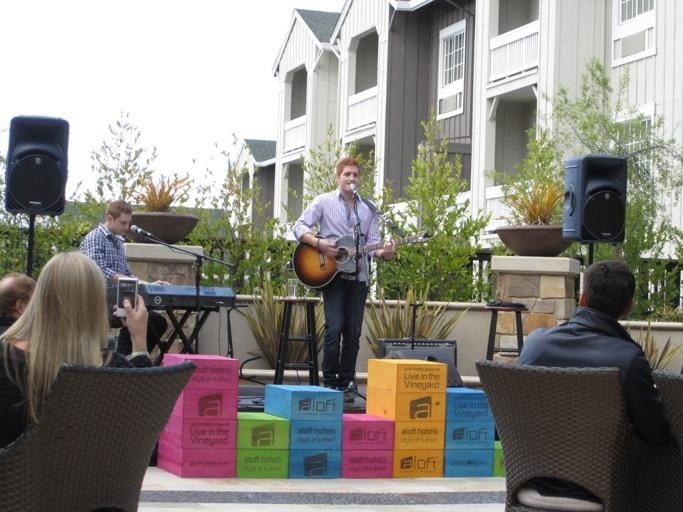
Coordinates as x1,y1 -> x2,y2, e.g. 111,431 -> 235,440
126,175 -> 200,244
495,183 -> 574,257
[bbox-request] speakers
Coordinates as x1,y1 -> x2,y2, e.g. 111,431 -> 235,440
562,154 -> 628,244
4,116 -> 69,217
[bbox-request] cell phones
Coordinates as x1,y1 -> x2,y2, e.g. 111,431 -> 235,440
117,277 -> 137,317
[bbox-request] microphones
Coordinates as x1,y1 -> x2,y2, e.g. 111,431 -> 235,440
349,183 -> 363,206
204,300 -> 225,305
130,225 -> 165,245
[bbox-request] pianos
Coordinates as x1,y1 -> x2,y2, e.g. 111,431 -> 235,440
115,283 -> 236,308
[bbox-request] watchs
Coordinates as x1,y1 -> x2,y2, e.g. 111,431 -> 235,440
315,238 -> 321,250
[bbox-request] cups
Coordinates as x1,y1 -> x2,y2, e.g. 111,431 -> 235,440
287,278 -> 299,299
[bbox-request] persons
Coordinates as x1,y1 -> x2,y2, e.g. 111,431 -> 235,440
517,260 -> 681,511
0,252 -> 156,456
0,271 -> 36,341
75,197 -> 168,371
293,157 -> 398,404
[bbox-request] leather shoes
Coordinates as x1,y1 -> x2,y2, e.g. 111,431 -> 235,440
325,384 -> 336,389
341,389 -> 355,403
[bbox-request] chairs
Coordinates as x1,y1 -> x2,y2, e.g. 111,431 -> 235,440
652,370 -> 683,455
475,359 -> 683,512
0,362 -> 197,512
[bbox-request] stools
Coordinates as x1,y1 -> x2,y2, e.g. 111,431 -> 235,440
274,297 -> 319,386
485,306 -> 529,361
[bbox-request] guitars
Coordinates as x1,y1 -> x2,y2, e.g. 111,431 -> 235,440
292,231 -> 434,288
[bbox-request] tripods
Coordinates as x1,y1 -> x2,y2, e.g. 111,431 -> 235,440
341,194 -> 368,401
